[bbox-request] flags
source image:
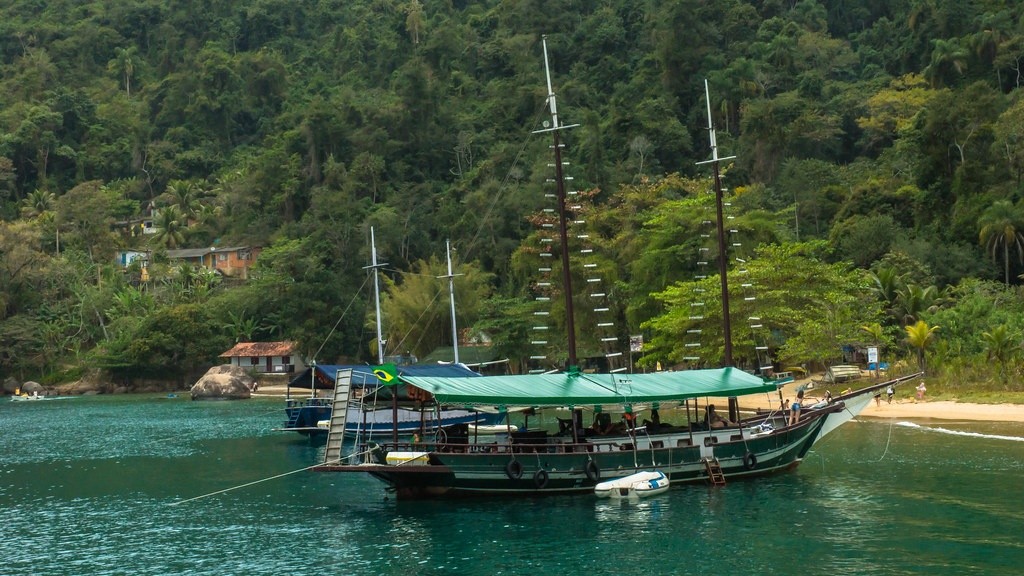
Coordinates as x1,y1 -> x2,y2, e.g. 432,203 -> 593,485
372,361 -> 404,389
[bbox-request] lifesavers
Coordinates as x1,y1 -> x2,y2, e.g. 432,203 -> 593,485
506,460 -> 523,479
535,470 -> 548,488
586,461 -> 601,481
743,453 -> 757,470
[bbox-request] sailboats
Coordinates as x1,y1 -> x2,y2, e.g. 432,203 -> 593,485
313,30 -> 924,496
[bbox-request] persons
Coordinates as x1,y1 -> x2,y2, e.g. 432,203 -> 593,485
789,390 -> 817,425
779,398 -> 789,410
22,392 -> 28,396
32,388 -> 38,395
887,385 -> 893,404
917,379 -> 925,400
14,386 -> 20,396
592,413 -> 616,436
874,383 -> 881,408
704,404 -> 731,427
651,409 -> 660,425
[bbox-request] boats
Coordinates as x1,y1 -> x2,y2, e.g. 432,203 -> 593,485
593,470 -> 671,499
270,225 -> 511,439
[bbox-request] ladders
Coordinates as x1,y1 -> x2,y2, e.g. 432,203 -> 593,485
286,408 -> 302,428
704,457 -> 726,486
